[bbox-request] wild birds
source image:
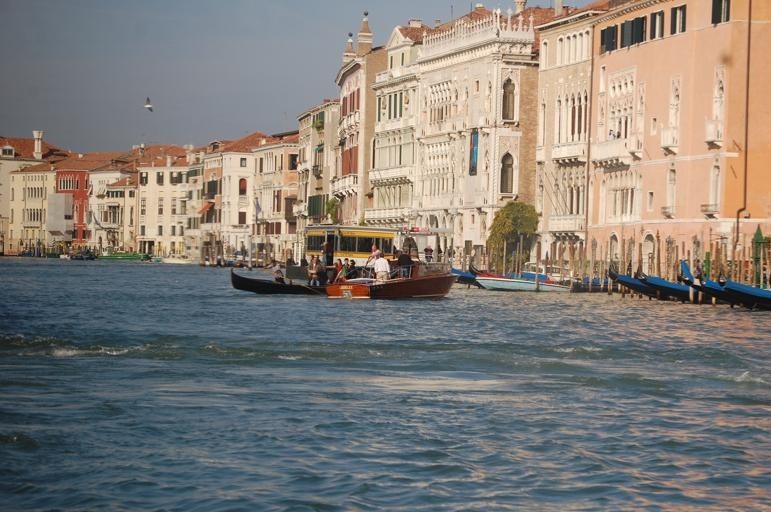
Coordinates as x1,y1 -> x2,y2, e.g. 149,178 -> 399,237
92,215 -> 104,230
143,96 -> 154,113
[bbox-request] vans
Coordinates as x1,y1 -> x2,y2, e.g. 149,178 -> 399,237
524,260 -> 573,284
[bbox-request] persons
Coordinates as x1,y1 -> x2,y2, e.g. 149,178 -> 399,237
374,253 -> 392,285
337,258 -> 343,274
339,260 -> 357,283
367,244 -> 382,262
309,255 -> 323,287
397,248 -> 415,279
341,258 -> 350,276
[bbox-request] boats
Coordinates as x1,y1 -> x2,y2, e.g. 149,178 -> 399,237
452,268 -> 574,293
231,263 -> 459,302
608,257 -> 771,309
